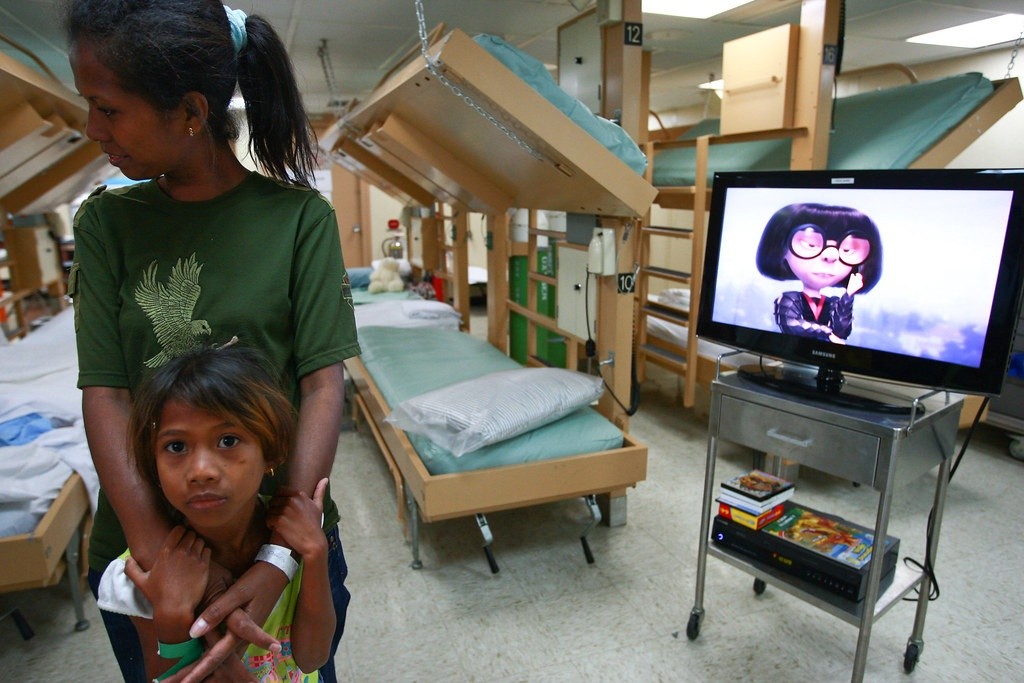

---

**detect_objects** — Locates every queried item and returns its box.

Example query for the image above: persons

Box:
[100,348,335,682]
[63,3,358,681]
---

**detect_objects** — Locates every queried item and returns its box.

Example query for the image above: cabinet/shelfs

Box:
[686,350,969,683]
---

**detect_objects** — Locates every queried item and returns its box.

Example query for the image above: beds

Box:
[308,21,661,575]
[638,61,1024,476]
[0,32,126,644]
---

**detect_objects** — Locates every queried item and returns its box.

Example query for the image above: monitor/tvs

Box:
[697,169,1024,415]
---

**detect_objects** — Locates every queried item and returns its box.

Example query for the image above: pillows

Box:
[345,268,375,289]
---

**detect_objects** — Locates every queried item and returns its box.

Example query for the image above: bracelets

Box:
[153,637,203,682]
[255,544,300,582]
